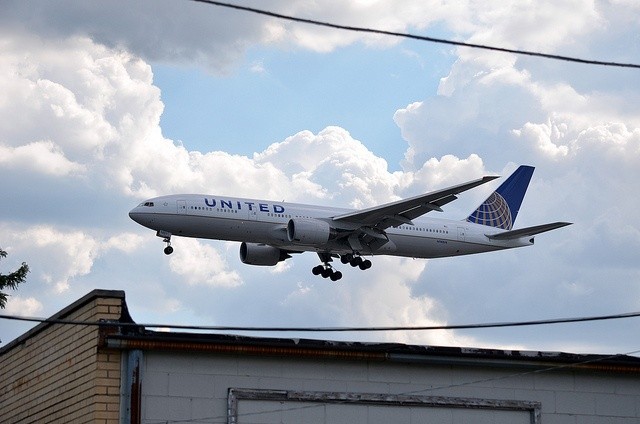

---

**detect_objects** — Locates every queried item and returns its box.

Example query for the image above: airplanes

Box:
[129,163,574,281]
